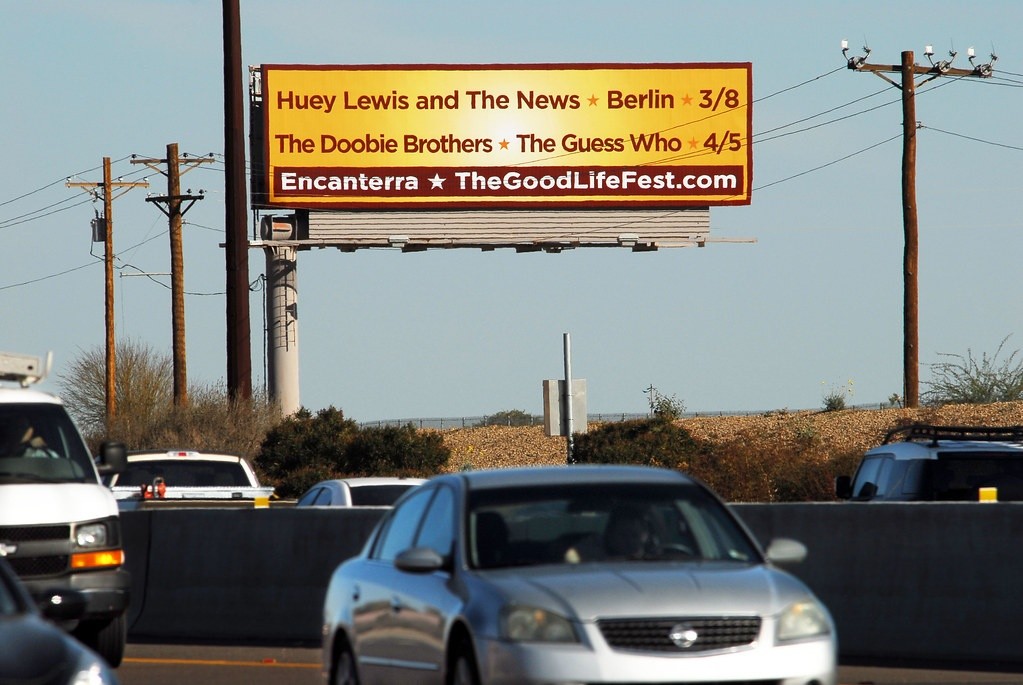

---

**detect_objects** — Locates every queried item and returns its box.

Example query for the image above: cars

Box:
[93,449,276,502]
[296,477,431,509]
[324,466,839,685]
[0,553,121,685]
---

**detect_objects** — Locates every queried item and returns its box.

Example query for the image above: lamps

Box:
[617,233,639,247]
[387,235,410,249]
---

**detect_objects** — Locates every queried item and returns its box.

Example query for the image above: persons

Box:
[0,414,59,460]
[603,506,651,560]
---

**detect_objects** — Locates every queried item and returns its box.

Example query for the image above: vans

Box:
[0,349,132,670]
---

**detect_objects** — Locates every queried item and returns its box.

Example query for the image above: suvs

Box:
[833,422,1023,502]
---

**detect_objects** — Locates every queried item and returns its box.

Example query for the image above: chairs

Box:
[602,509,657,554]
[481,506,515,562]
